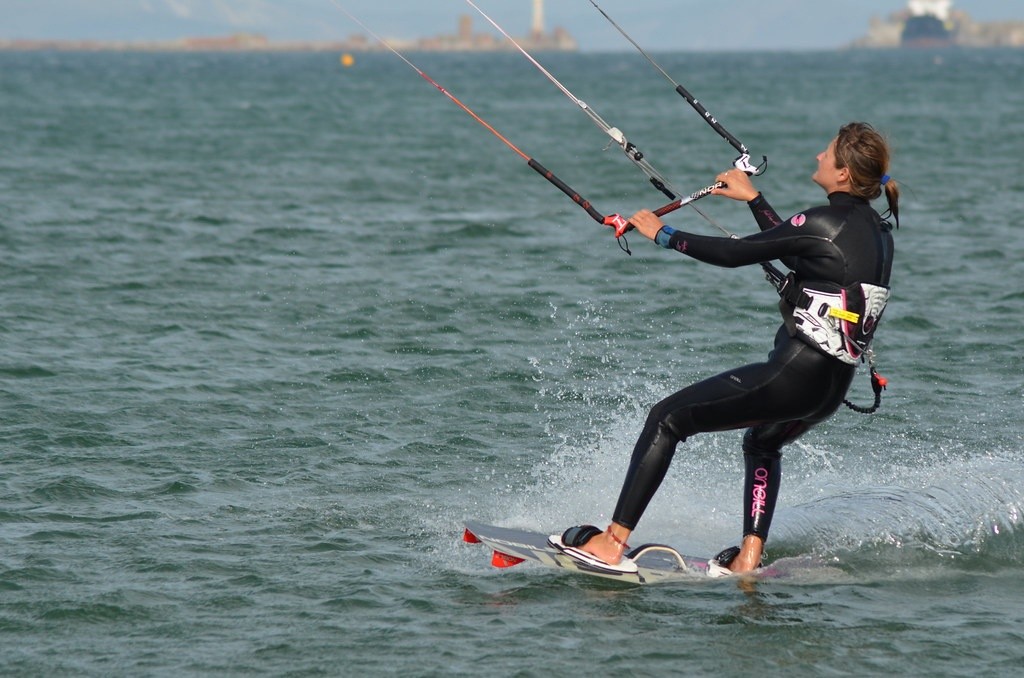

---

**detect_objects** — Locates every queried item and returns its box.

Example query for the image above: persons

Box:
[561,122,900,576]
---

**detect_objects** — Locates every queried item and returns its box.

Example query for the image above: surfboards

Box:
[462,518,765,590]
